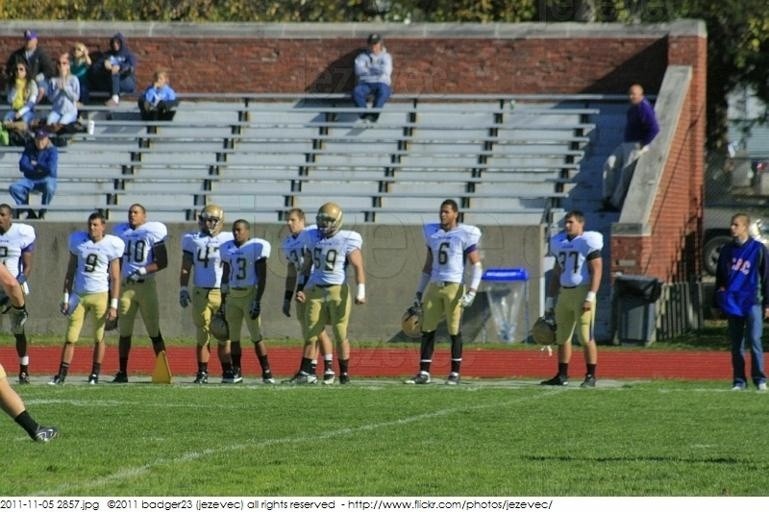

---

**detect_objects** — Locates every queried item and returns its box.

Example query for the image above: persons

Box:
[45,208,123,387]
[409,198,483,386]
[2,28,175,217]
[112,204,171,387]
[544,213,606,387]
[178,205,365,393]
[596,85,661,215]
[356,35,393,121]
[1,206,35,386]
[714,215,766,391]
[0,267,61,446]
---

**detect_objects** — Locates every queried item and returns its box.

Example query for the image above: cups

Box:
[86,120,96,136]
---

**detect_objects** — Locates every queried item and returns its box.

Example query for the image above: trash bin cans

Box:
[614,276,664,349]
[480,267,529,344]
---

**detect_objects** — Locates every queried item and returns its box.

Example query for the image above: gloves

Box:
[127,270,141,284]
[461,291,476,309]
[180,290,192,307]
[283,296,292,316]
[249,302,261,320]
[10,304,29,331]
[219,303,226,317]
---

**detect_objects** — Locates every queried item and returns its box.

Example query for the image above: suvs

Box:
[700,204,769,276]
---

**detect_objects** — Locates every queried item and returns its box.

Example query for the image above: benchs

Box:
[0,92,657,225]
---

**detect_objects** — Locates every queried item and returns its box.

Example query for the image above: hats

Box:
[24,28,38,40]
[34,129,48,139]
[366,32,381,44]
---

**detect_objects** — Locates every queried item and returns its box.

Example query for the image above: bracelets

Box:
[546,295,553,311]
[62,293,69,305]
[111,297,119,309]
[416,271,429,295]
[584,289,596,303]
[138,265,147,274]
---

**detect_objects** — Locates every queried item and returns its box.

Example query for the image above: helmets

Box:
[211,306,229,342]
[534,317,556,344]
[316,201,343,237]
[401,306,422,338]
[198,206,224,237]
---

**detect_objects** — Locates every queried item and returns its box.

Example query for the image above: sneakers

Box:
[757,383,768,391]
[19,374,30,384]
[222,368,242,383]
[48,375,64,385]
[33,422,59,445]
[263,370,274,384]
[404,369,431,384]
[581,375,596,388]
[540,373,568,386]
[113,372,128,383]
[733,382,745,391]
[445,371,461,384]
[281,370,350,385]
[89,375,98,385]
[194,369,208,384]
